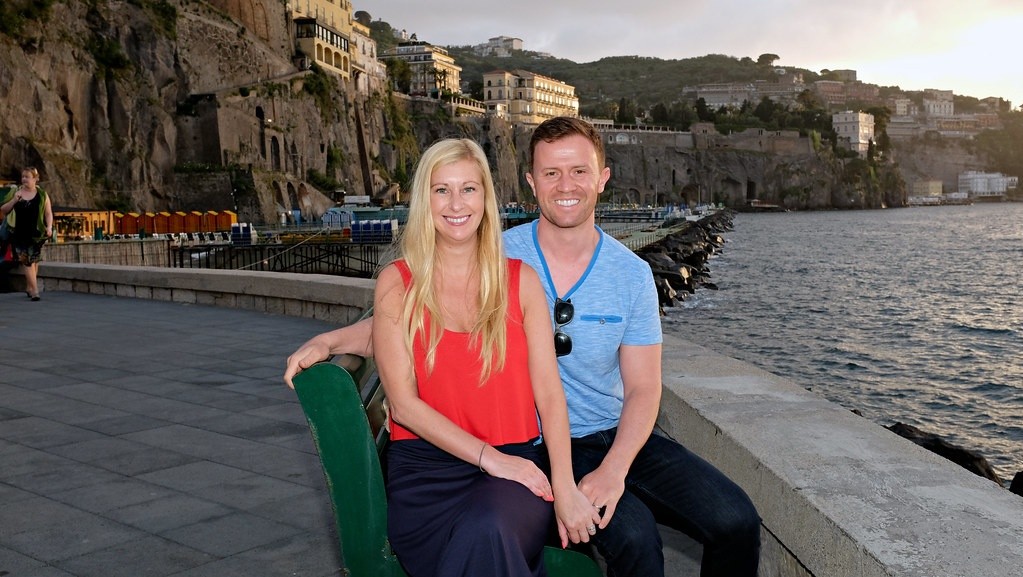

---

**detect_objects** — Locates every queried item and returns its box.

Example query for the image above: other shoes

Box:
[26,289,40,301]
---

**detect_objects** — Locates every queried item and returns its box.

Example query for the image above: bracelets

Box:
[479,442,490,474]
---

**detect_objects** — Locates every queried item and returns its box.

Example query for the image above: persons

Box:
[282,115,763,577]
[0,166,54,302]
[371,136,600,577]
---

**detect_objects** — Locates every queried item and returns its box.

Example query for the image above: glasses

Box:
[553,297,575,358]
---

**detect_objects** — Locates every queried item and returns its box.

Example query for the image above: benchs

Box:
[291,306,603,577]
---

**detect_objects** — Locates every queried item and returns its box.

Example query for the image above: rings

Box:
[593,504,602,511]
[587,526,596,531]
